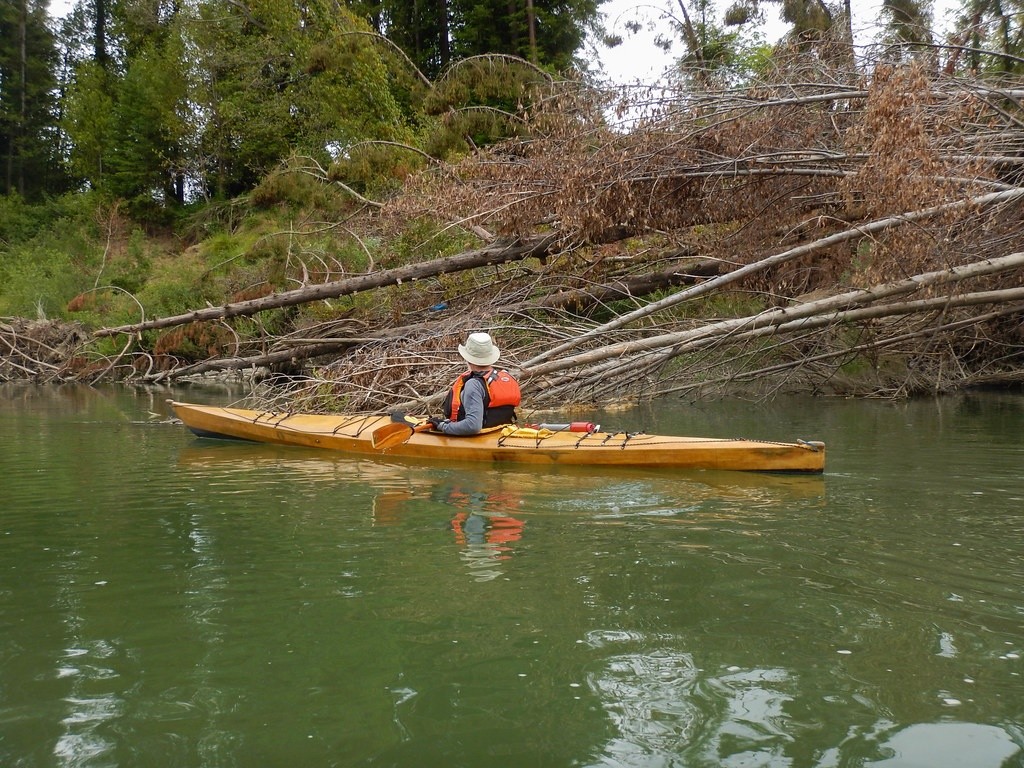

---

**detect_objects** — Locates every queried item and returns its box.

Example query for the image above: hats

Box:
[458,332,501,366]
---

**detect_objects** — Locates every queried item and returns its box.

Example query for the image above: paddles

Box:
[370,419,450,450]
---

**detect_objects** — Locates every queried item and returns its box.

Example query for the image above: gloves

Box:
[426,417,444,431]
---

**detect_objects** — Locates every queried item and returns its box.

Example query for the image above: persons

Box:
[425,333,521,435]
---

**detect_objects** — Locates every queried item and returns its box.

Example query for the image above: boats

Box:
[166,398,826,475]
